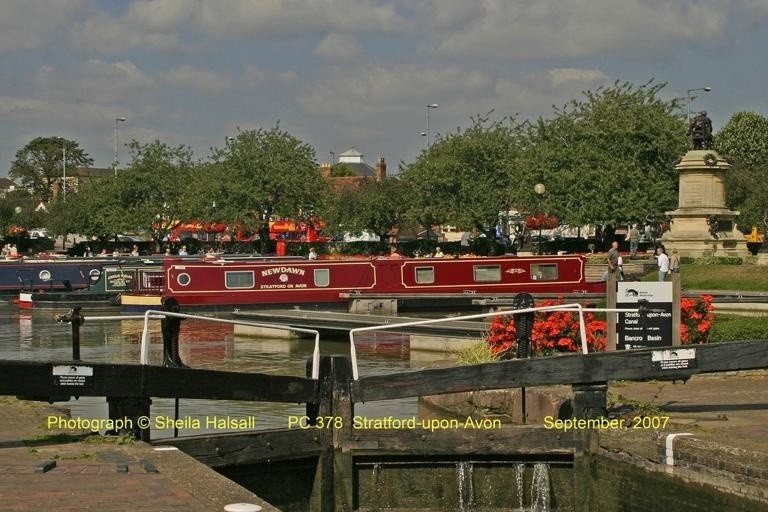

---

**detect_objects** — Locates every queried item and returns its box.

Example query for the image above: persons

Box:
[656,247,669,282]
[686,111,712,136]
[607,242,619,272]
[1,243,18,257]
[97,244,120,257]
[460,229,474,253]
[178,245,188,256]
[164,248,172,256]
[670,247,680,272]
[131,244,140,256]
[83,246,93,257]
[625,223,639,255]
[390,245,401,257]
[433,246,444,257]
[308,247,317,259]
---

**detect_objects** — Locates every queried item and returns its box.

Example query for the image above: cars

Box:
[343,228,380,243]
[532,234,652,254]
[29,230,56,243]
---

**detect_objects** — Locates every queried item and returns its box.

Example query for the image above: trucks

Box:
[468,210,528,252]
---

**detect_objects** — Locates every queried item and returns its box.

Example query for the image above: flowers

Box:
[485,295,608,355]
[680,294,715,344]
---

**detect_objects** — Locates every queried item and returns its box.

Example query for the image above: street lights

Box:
[211,200,217,253]
[686,87,711,148]
[14,206,23,254]
[420,103,438,240]
[57,136,66,250]
[228,136,235,147]
[114,117,126,180]
[534,182,545,255]
